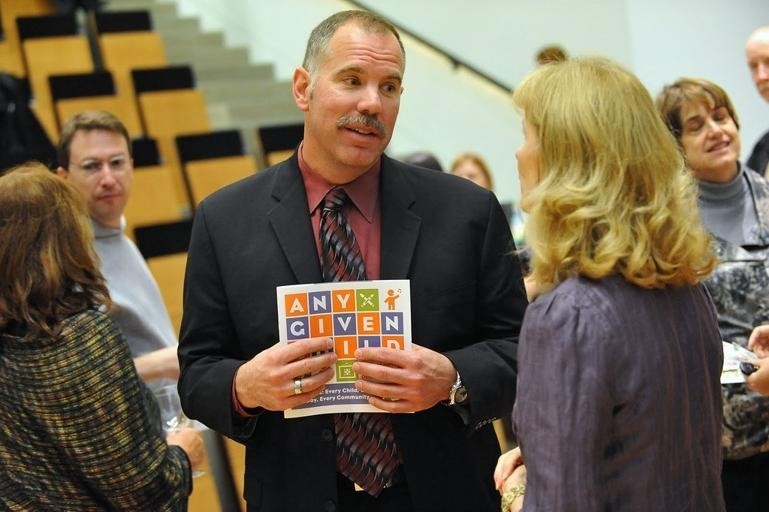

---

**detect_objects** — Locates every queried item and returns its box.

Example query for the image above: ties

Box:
[316,187,401,498]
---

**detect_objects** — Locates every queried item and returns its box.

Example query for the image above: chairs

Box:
[86,5,153,65]
[45,69,117,134]
[133,217,193,259]
[258,122,304,168]
[129,137,164,172]
[130,62,197,136]
[14,11,80,80]
[173,127,250,215]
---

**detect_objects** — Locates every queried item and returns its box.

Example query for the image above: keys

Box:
[740,362,760,375]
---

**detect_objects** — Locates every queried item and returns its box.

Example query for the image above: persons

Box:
[174,7,533,512]
[738,324,769,400]
[52,109,212,436]
[0,158,208,510]
[489,54,732,511]
[649,75,768,512]
[448,154,493,193]
[741,25,768,187]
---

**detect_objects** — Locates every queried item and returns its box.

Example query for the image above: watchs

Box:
[443,370,469,408]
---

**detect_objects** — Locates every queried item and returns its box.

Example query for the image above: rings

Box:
[294,378,303,395]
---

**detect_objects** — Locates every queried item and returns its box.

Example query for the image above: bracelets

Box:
[500,482,527,511]
